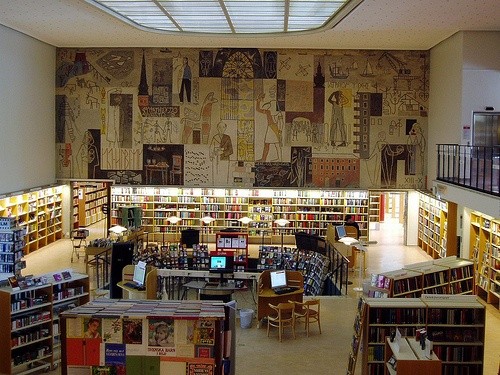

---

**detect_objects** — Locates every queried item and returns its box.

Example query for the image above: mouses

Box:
[138,288,143,291]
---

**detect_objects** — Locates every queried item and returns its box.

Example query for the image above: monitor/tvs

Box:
[133,265,147,290]
[181,230,199,248]
[336,225,346,238]
[270,270,288,291]
[211,256,227,269]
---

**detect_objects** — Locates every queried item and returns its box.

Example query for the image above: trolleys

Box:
[70,230,87,263]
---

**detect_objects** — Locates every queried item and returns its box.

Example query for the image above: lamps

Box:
[165,216,182,224]
[237,216,253,224]
[199,216,215,223]
[274,219,290,227]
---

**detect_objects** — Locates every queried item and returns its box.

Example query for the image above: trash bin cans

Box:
[240,309,255,329]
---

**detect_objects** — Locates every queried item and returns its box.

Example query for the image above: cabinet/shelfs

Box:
[0,181,500,375]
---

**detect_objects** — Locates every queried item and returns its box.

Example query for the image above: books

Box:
[427,308,484,375]
[73,181,107,228]
[110,186,369,237]
[417,192,447,258]
[368,308,425,375]
[345,296,366,375]
[9,272,84,367]
[66,299,225,375]
[0,215,26,272]
[0,183,62,243]
[366,265,472,298]
[370,192,380,222]
[471,215,500,296]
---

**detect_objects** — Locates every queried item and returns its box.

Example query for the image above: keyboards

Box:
[275,287,294,295]
[124,283,140,289]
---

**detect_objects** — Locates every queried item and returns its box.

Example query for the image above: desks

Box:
[157,268,260,303]
[117,280,146,292]
[259,287,304,297]
[183,281,248,301]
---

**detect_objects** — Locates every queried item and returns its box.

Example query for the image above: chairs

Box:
[266,303,296,342]
[287,299,322,338]
